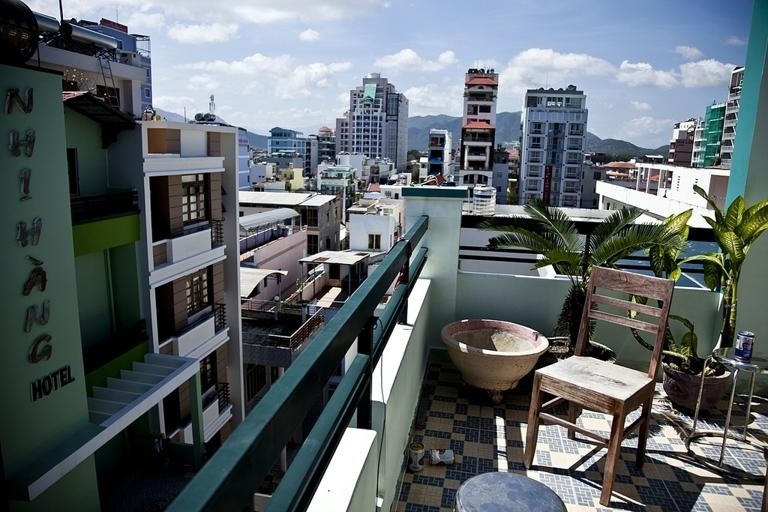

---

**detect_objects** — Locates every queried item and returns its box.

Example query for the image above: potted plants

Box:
[485,194,675,407]
[658,185,768,411]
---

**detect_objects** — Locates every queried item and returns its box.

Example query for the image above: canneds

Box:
[734,329,755,363]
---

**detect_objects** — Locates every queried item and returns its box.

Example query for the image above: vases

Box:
[442,317,550,404]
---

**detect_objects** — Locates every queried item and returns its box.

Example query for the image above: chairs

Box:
[518,262,675,506]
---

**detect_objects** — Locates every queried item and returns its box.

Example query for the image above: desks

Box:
[690,345,767,468]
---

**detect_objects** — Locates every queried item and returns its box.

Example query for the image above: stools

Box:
[454,471,568,512]
